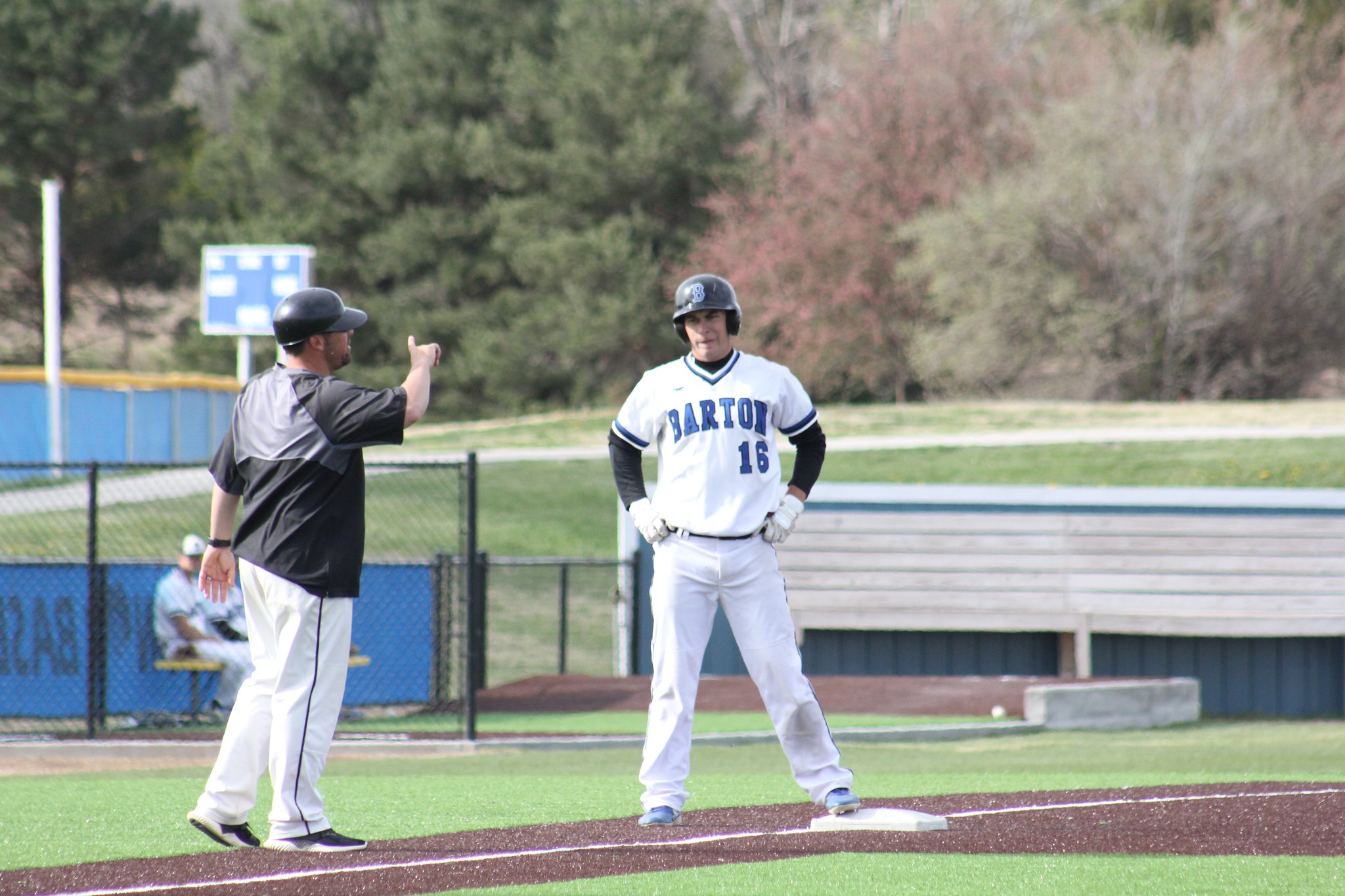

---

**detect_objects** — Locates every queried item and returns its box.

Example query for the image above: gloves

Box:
[629,498,675,544]
[753,495,805,543]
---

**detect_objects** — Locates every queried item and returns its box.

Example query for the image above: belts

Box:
[667,527,766,540]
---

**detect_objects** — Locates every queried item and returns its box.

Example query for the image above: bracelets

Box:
[207,535,231,548]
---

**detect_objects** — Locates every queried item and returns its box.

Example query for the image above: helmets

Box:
[673,274,742,345]
[272,289,368,345]
[178,535,205,555]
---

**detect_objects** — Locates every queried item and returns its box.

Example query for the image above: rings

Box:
[207,575,212,581]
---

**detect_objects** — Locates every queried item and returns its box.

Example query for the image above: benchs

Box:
[153,654,370,728]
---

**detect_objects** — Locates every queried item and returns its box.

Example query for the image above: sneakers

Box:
[825,788,861,815]
[187,811,260,847]
[263,829,369,852]
[637,806,682,827]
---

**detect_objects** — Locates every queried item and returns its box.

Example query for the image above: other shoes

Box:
[338,708,364,723]
[138,712,177,728]
[204,700,232,721]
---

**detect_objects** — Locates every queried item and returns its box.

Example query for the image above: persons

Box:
[188,286,441,852]
[154,535,254,724]
[608,274,862,827]
